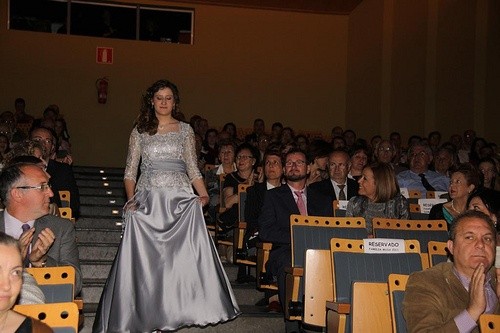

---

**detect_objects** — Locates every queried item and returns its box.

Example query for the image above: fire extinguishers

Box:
[96,76,109,104]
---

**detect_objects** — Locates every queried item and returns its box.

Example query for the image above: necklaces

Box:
[452,200,462,214]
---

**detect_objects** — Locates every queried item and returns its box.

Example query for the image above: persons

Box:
[0,98,83,333]
[176,112,500,333]
[92,80,243,333]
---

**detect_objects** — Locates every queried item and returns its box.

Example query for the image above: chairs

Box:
[200,165,500,333]
[11,189,83,333]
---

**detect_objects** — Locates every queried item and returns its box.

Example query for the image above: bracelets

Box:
[40,259,46,267]
